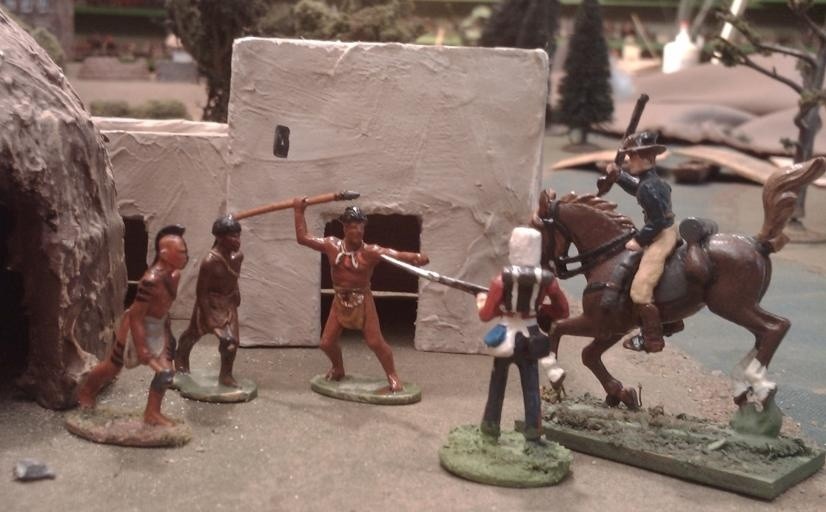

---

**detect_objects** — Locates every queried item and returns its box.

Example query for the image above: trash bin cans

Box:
[621,27,641,59]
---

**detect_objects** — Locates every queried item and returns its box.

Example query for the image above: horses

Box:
[538,156,825,411]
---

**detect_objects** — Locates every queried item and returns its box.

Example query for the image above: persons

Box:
[175,216,253,389]
[604,132,687,352]
[466,226,570,447]
[73,224,189,430]
[291,195,430,394]
